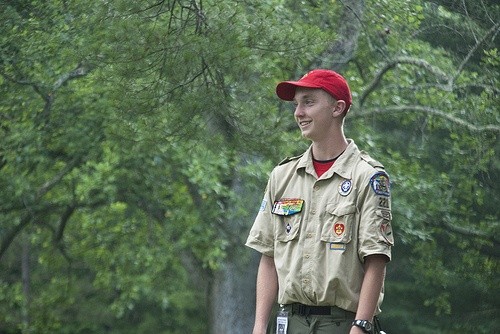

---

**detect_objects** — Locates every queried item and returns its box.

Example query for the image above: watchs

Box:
[352,319,372,333]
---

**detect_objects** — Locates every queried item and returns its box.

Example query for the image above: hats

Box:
[276,69,352,112]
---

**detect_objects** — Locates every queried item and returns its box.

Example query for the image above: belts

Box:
[292,302,332,316]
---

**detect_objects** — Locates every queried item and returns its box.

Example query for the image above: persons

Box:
[245,69,394,334]
